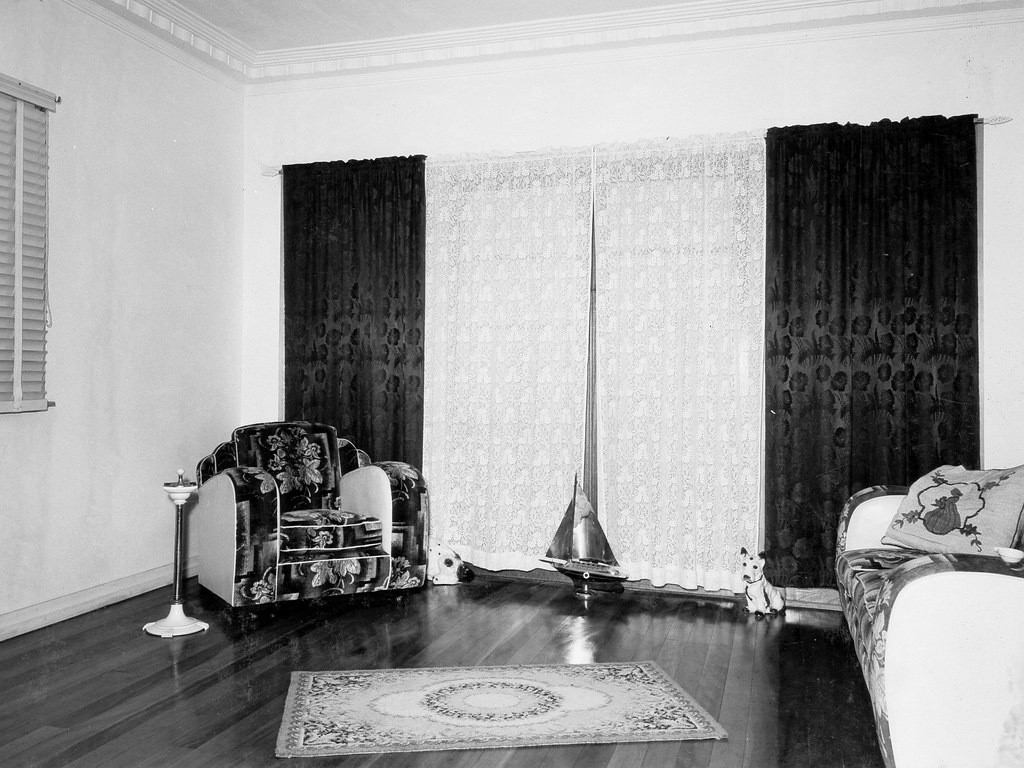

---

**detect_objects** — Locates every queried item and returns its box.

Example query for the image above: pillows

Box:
[231,420,344,515]
[881,465,1024,557]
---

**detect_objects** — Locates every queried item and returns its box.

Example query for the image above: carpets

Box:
[274,659,731,759]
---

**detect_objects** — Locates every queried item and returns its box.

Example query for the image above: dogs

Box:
[740,546,787,618]
[426,537,476,585]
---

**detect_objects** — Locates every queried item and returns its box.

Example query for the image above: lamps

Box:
[139,467,209,637]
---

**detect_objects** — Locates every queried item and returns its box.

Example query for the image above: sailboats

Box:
[538,472,629,592]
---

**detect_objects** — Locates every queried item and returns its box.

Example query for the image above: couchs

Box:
[835,463,1024,768]
[196,420,428,606]
